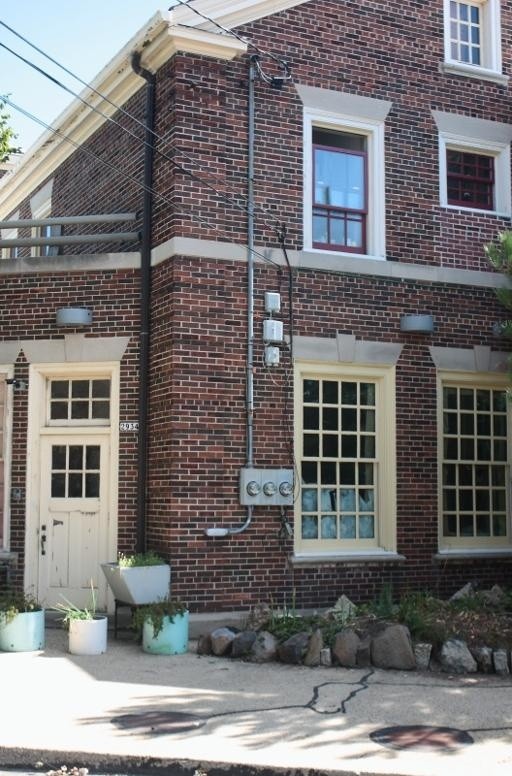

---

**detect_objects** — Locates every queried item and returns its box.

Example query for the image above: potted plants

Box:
[99,550,171,606]
[124,592,189,655]
[51,575,108,656]
[0,583,47,652]
